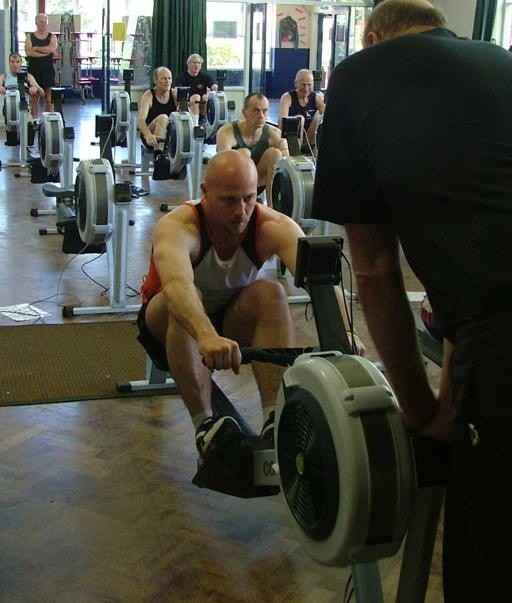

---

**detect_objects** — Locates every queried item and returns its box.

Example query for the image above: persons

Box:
[310,0,512,603]
[25,13,57,119]
[136,67,180,161]
[0,53,45,124]
[216,93,290,209]
[134,149,366,500]
[277,68,326,148]
[177,53,218,127]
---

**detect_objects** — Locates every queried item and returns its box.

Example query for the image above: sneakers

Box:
[195,414,243,457]
[125,182,148,199]
[259,413,280,444]
[153,150,168,164]
